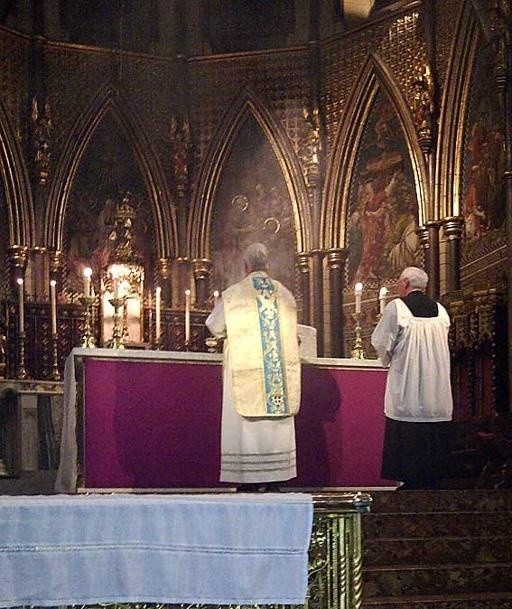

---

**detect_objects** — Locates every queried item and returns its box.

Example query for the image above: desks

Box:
[59,347,403,490]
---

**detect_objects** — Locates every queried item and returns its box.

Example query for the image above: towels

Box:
[0,492,313,609]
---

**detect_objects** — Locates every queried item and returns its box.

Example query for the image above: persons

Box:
[205,243,302,492]
[370,267,454,490]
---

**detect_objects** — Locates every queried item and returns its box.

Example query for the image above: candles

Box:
[379,287,387,314]
[50,280,57,334]
[354,282,363,313]
[17,278,24,332]
[184,290,191,341]
[114,277,121,298]
[83,268,93,298]
[155,287,162,338]
[214,291,219,308]
